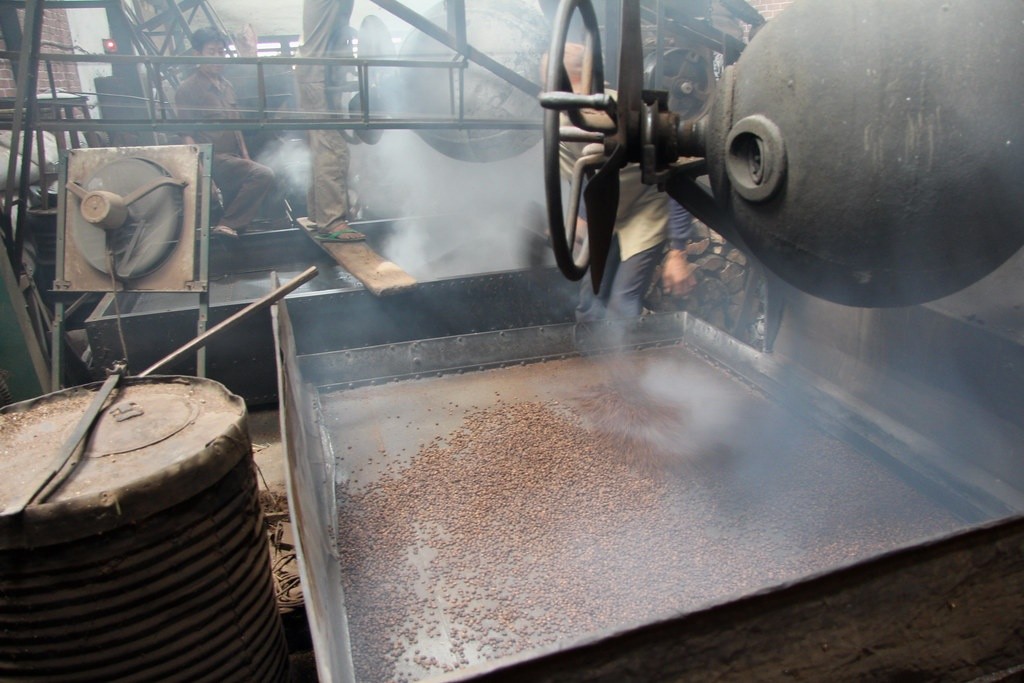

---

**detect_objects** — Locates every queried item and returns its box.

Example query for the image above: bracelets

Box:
[670,251,683,258]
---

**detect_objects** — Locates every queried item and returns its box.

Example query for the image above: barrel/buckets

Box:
[1,374,288,683]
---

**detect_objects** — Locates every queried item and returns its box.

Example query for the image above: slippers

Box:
[315,225,368,242]
[307,222,317,228]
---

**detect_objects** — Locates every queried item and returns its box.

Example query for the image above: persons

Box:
[173,27,276,241]
[542,44,695,323]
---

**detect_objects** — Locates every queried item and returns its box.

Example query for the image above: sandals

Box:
[213,224,239,240]
